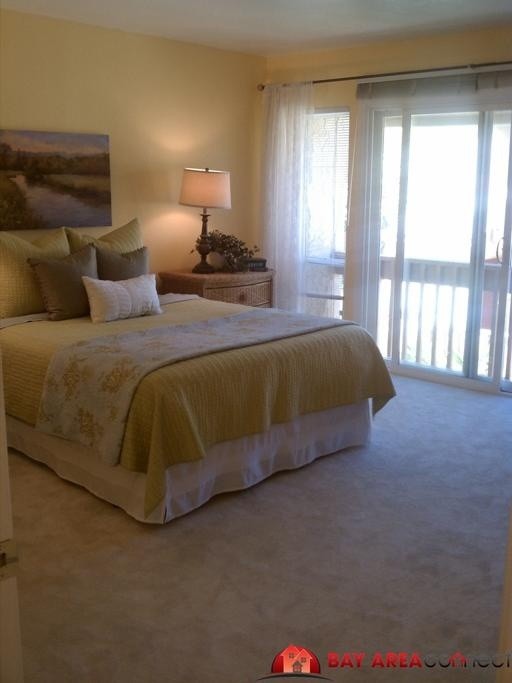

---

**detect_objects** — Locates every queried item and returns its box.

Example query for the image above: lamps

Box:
[178,168,232,273]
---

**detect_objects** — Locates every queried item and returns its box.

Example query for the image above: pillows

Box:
[0,217,162,321]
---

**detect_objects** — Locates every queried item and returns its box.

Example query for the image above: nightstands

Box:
[159,269,276,308]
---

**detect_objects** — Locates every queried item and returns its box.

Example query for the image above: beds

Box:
[0,230,398,526]
